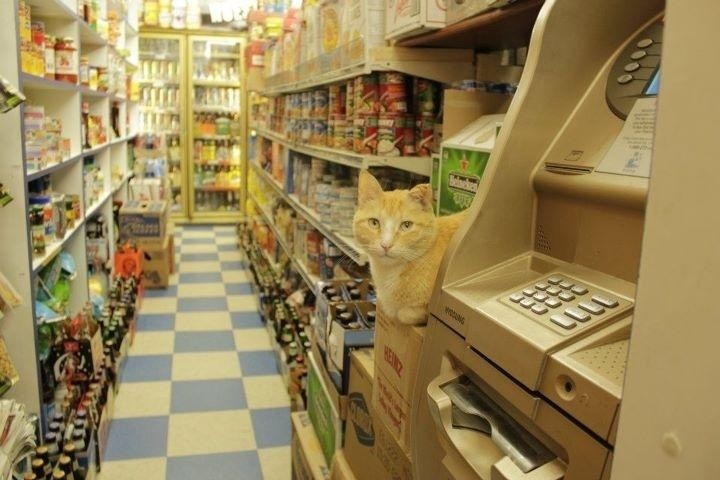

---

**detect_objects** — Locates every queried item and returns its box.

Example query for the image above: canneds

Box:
[45,34,56,81]
[28,206,45,255]
[264,72,441,157]
[314,174,359,237]
[54,38,77,83]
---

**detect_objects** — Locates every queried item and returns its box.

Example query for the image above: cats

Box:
[345,167,472,326]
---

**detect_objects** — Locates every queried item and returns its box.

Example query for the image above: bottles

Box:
[139,39,239,211]
[270,276,376,384]
[25,273,144,480]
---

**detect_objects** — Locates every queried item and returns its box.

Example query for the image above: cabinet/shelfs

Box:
[0,0,142,480]
[240,32,473,412]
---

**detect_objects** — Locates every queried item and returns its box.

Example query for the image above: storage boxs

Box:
[288,276,428,480]
[119,194,179,294]
